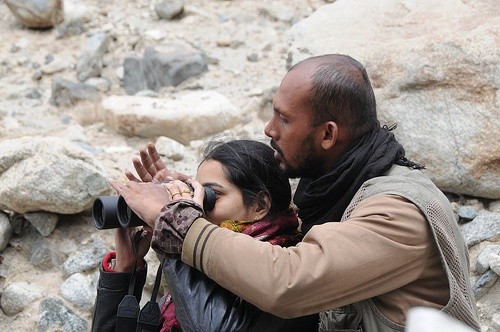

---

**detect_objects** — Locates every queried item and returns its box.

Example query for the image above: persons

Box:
[109,52,480,332]
[89,139,320,332]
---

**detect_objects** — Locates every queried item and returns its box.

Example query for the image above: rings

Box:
[180,191,191,196]
[172,192,181,199]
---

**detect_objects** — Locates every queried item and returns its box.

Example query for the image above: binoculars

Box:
[91,183,218,232]
